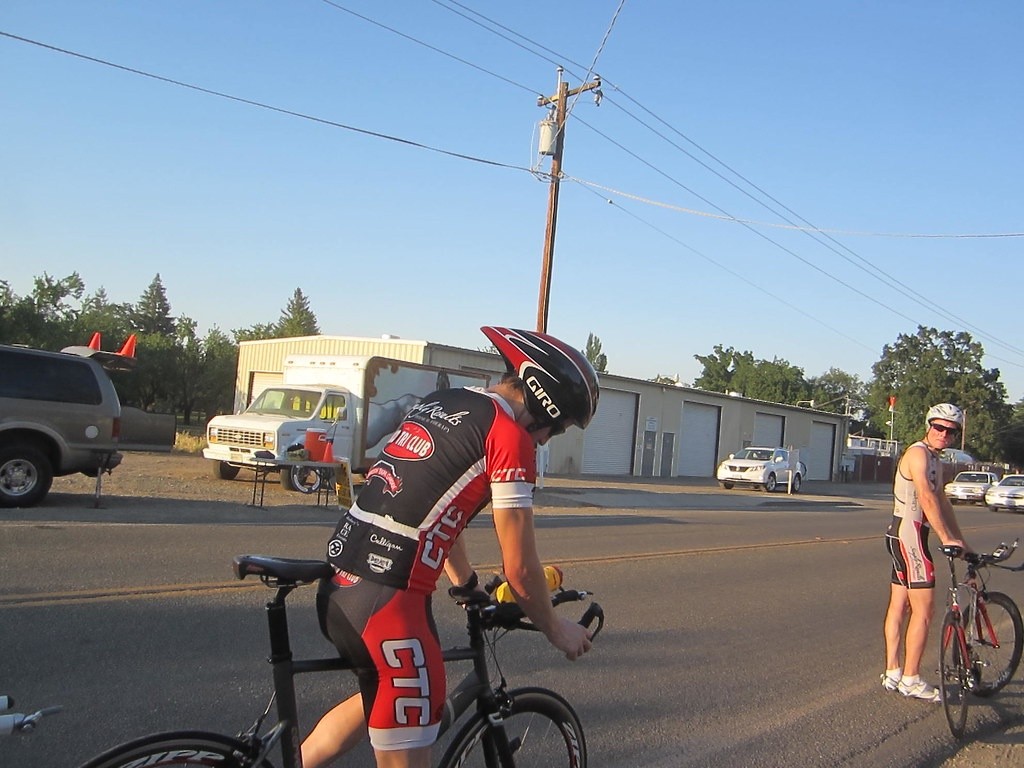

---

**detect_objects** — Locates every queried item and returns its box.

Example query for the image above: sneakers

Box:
[881,672,903,690]
[897,678,949,702]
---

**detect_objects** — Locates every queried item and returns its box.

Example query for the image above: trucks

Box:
[202,354,492,492]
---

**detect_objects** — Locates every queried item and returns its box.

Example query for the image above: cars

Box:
[944,471,998,505]
[984,475,1024,512]
[717,446,802,493]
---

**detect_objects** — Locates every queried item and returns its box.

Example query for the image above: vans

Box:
[0,345,138,509]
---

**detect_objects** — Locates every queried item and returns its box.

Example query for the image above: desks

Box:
[249,457,341,510]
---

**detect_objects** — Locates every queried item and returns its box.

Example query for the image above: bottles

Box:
[497,566,563,602]
[281,444,286,459]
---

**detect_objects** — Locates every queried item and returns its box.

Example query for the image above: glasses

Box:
[549,418,565,437]
[930,423,957,436]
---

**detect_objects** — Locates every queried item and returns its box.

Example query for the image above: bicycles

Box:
[80,555,605,768]
[937,538,1024,739]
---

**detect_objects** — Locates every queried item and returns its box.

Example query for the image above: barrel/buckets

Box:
[304,428,327,461]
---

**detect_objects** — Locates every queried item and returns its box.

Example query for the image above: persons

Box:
[879,402,975,702]
[299,324,601,767]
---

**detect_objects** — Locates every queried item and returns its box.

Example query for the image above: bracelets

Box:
[448,570,478,597]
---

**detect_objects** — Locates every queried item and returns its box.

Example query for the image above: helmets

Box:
[925,403,962,433]
[480,323,599,430]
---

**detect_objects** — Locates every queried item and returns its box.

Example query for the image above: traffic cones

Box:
[88,333,101,351]
[322,442,335,463]
[116,334,137,357]
